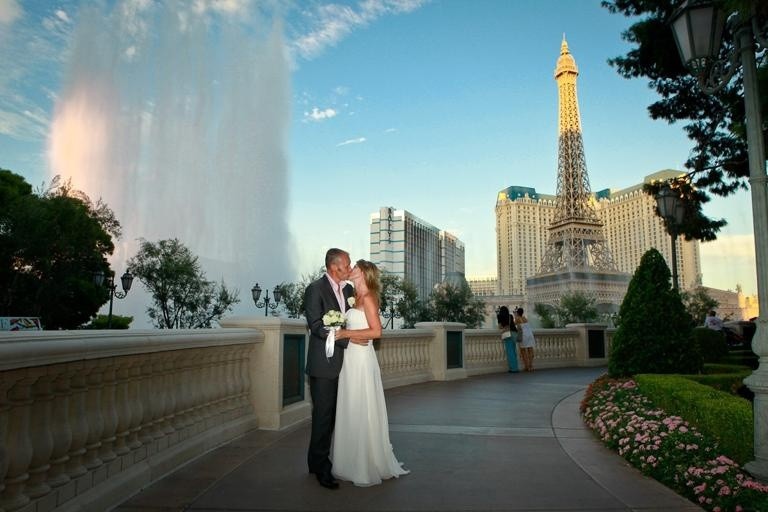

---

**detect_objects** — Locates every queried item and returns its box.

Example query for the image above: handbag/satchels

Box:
[501,331,512,340]
[517,325,523,342]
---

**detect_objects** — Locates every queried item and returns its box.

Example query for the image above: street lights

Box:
[667,1,768,481]
[252,282,283,316]
[655,185,685,292]
[611,312,621,327]
[380,298,406,329]
[495,305,518,317]
[93,268,134,328]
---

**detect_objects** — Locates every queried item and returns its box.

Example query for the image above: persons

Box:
[497,305,519,372]
[706,310,729,349]
[326,259,412,489]
[512,306,536,371]
[302,246,369,490]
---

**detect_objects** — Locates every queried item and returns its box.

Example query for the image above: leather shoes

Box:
[316,474,339,490]
